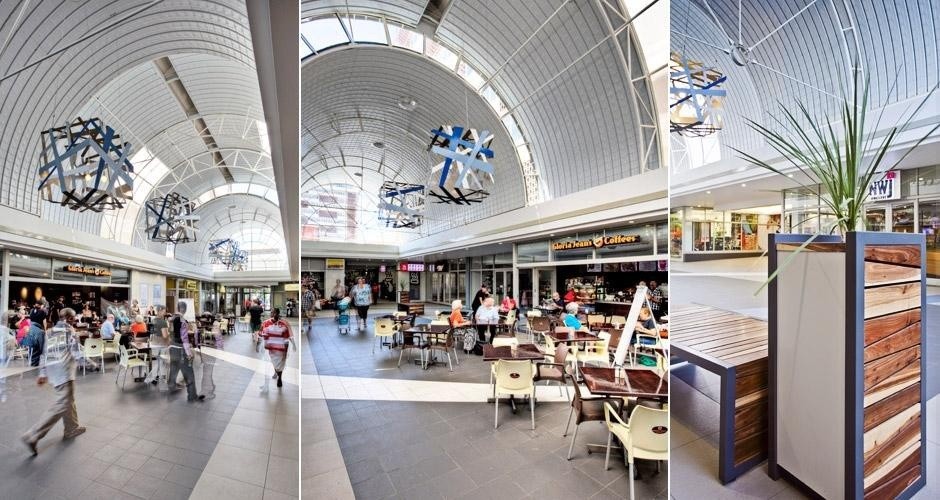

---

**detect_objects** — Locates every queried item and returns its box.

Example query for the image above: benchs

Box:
[668,302,769,487]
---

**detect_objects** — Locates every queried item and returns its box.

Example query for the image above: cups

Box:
[615,323,619,329]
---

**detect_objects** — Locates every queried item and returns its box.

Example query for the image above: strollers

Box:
[335,304,351,336]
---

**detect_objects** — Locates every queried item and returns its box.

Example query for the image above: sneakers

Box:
[276,377,282,387]
[196,394,205,400]
[22,439,39,455]
[64,426,86,439]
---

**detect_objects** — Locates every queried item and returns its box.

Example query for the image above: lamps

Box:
[208,202,249,273]
[146,131,199,246]
[38,0,134,213]
[670,0,726,137]
[377,100,425,231]
[427,34,495,204]
[0,301,256,390]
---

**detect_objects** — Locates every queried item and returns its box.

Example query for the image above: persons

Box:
[0,276,668,456]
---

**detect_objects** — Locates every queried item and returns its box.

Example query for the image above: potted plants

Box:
[723,53,939,282]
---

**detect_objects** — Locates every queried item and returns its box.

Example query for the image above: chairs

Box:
[376,296,668,500]
